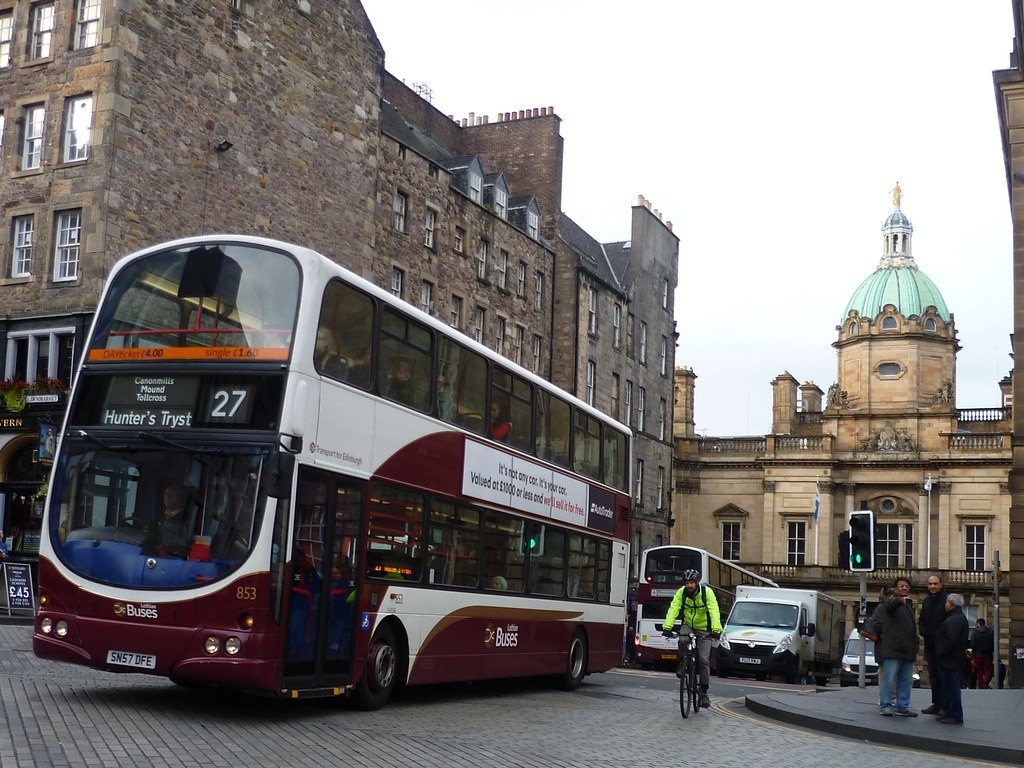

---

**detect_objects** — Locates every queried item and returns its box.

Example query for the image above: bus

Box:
[636,546,779,671]
[31,233,635,709]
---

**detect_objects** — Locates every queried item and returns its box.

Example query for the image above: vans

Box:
[840,626,879,686]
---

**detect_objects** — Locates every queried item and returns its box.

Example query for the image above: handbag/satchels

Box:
[861,619,882,641]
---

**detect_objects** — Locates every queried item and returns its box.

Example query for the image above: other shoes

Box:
[676,662,683,678]
[936,715,947,720]
[921,703,938,713]
[890,699,896,706]
[940,718,956,723]
[698,685,710,707]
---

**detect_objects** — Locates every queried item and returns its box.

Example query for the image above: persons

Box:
[870,586,897,706]
[933,594,969,725]
[880,578,919,717]
[919,574,950,715]
[889,181,903,208]
[961,651,1006,689]
[136,485,185,534]
[437,376,456,420]
[662,570,722,707]
[314,327,341,377]
[623,627,635,664]
[0,531,9,571]
[480,401,512,444]
[491,576,507,590]
[971,618,994,689]
[386,361,415,405]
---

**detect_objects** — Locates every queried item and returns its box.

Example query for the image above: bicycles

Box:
[662,631,720,719]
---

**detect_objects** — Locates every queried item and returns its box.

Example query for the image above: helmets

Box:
[686,570,700,582]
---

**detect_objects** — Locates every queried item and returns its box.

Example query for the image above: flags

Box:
[814,481,820,523]
[924,476,932,490]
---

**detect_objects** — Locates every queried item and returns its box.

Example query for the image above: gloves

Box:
[662,630,674,637]
[709,632,720,640]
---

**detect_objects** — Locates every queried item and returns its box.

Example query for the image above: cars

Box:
[911,663,921,688]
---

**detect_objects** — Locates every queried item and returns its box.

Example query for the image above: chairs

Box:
[453,412,599,480]
[333,356,350,382]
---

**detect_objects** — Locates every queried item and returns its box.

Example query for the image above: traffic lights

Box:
[849,511,875,572]
[518,518,544,556]
[837,532,849,570]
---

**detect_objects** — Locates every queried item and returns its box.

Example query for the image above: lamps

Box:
[214,140,233,153]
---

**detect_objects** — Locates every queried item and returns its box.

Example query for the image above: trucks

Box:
[716,585,846,685]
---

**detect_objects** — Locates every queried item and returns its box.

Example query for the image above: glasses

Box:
[492,408,497,411]
[929,582,938,585]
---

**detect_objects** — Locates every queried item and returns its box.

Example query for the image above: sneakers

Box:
[895,708,918,717]
[880,709,892,716]
